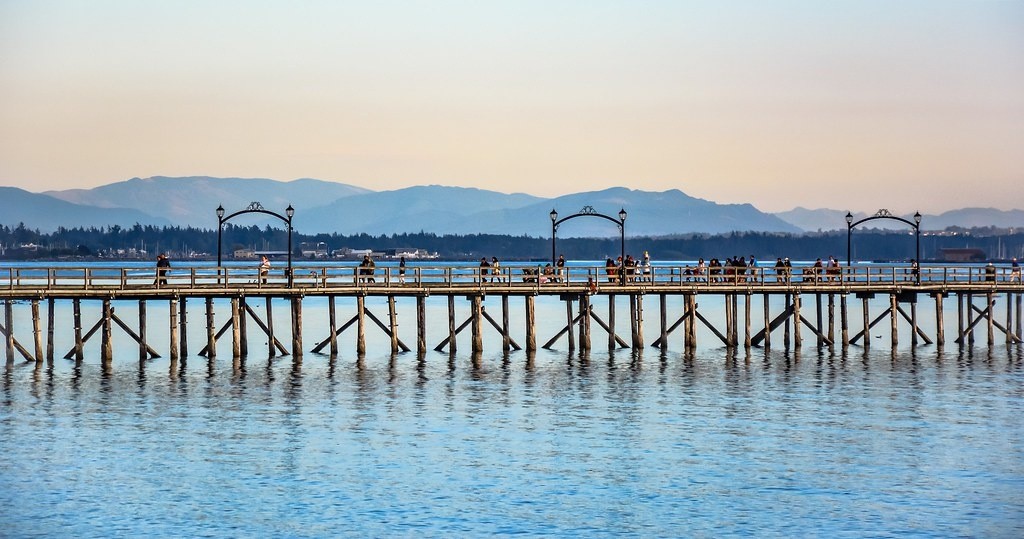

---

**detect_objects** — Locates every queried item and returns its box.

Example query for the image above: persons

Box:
[544,264,557,282]
[748,255,759,282]
[692,266,698,282]
[400,257,405,284]
[683,265,691,282]
[360,256,375,282]
[260,255,270,284]
[910,259,917,282]
[774,257,792,283]
[557,255,564,283]
[724,256,747,282]
[698,258,706,283]
[153,253,171,285]
[606,256,622,282]
[490,257,501,283]
[624,255,650,282]
[480,258,490,283]
[803,258,822,282]
[1010,257,1020,282]
[708,258,722,283]
[826,255,841,282]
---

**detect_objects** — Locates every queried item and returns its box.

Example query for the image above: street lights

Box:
[216,203,225,283]
[550,207,559,282]
[845,211,853,280]
[619,207,630,286]
[285,204,296,287]
[913,211,923,286]
